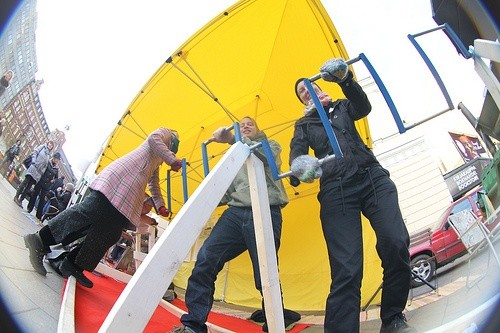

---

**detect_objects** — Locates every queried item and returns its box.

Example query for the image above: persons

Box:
[0,70,137,263]
[25,128,183,288]
[290,58,417,333]
[166,115,290,333]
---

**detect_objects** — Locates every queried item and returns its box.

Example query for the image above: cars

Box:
[406,184,498,287]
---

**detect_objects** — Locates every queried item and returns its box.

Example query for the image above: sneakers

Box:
[380,313,415,333]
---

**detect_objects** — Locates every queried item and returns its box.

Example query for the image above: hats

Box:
[53,152,61,159]
[66,183,74,191]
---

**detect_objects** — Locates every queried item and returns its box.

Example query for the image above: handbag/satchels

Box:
[23,155,32,170]
[46,241,84,278]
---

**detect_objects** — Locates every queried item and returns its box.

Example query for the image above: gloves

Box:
[158,206,169,216]
[171,159,181,172]
[290,155,323,184]
[319,57,349,82]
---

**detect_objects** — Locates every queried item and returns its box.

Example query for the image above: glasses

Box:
[299,81,315,97]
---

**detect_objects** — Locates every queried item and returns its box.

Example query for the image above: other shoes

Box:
[36,214,41,220]
[263,320,270,333]
[28,209,32,213]
[168,326,207,333]
[24,233,47,275]
[14,197,24,208]
[58,258,94,288]
